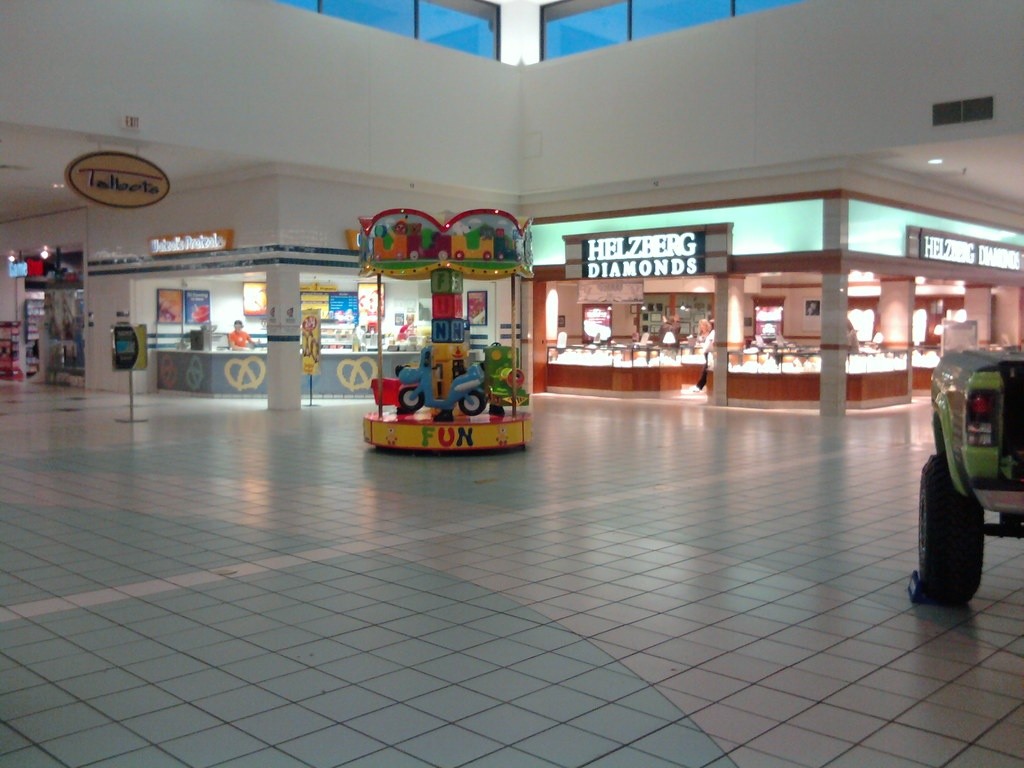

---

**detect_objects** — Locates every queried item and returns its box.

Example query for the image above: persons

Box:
[657,315,674,348]
[697,319,711,344]
[663,313,681,348]
[229,320,256,352]
[32,338,40,359]
[397,313,414,340]
[847,319,860,353]
[691,320,714,392]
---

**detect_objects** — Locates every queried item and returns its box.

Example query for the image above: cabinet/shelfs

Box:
[158,350,422,395]
[679,343,707,385]
[546,344,683,391]
[910,344,942,390]
[706,345,911,402]
